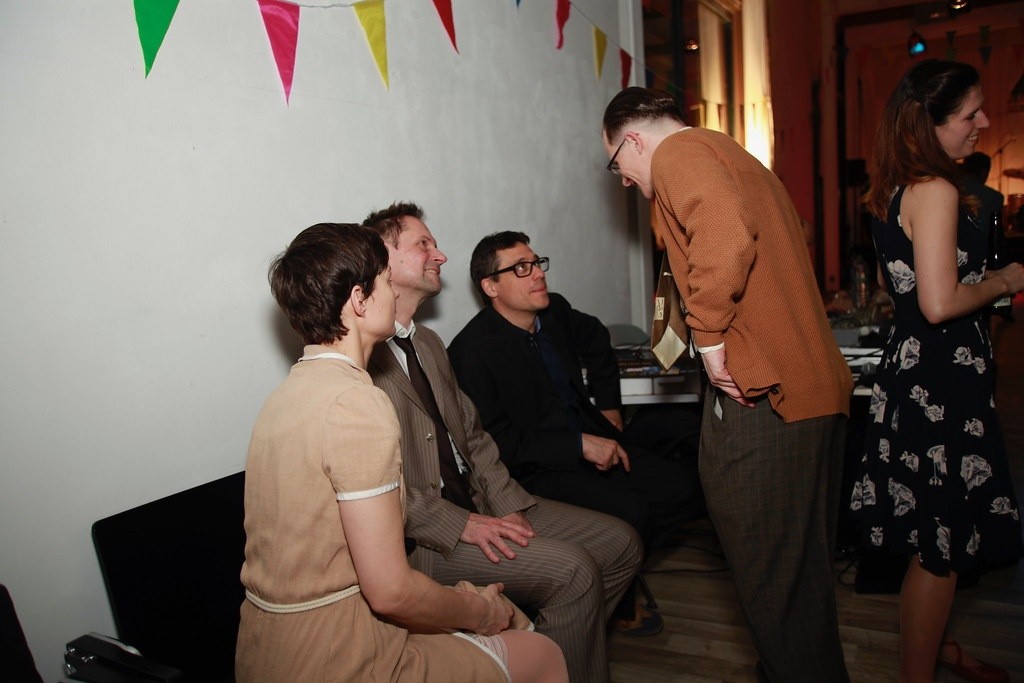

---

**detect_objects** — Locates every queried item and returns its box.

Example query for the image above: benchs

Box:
[64,470,246,683]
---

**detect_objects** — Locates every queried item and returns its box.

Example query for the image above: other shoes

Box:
[608,601,665,638]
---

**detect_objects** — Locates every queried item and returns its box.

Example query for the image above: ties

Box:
[392,332,483,515]
[651,244,688,373]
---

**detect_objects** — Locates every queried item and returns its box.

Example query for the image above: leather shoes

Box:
[937,639,1011,683]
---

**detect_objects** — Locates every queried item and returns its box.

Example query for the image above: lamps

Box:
[907,32,927,56]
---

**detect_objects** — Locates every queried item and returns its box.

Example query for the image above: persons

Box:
[446,231,664,636]
[603,87,853,683]
[846,58,1024,683]
[361,203,640,683]
[961,153,1003,265]
[234,223,569,683]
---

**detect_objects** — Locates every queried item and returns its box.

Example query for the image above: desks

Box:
[590,347,884,406]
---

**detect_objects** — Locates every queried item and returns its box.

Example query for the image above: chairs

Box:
[0,583,46,683]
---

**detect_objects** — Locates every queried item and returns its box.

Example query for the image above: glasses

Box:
[606,133,640,177]
[488,257,549,278]
